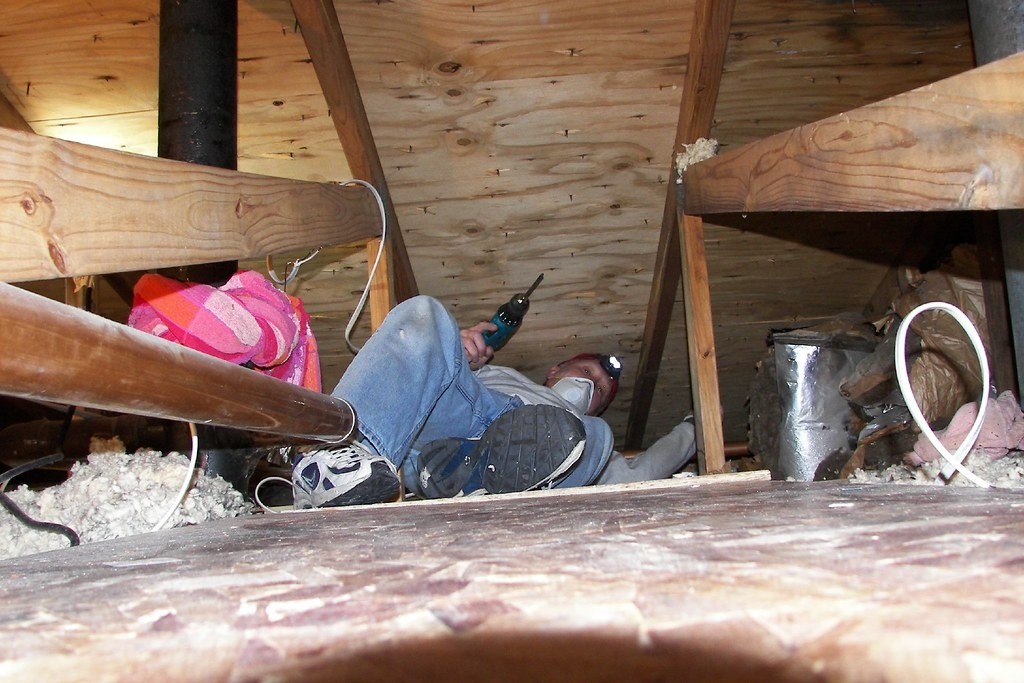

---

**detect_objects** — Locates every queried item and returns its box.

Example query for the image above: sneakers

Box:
[291,443,401,509]
[417,404,587,500]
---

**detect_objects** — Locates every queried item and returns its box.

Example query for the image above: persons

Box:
[291,296,701,509]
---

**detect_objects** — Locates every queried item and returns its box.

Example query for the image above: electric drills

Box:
[478,274,543,352]
